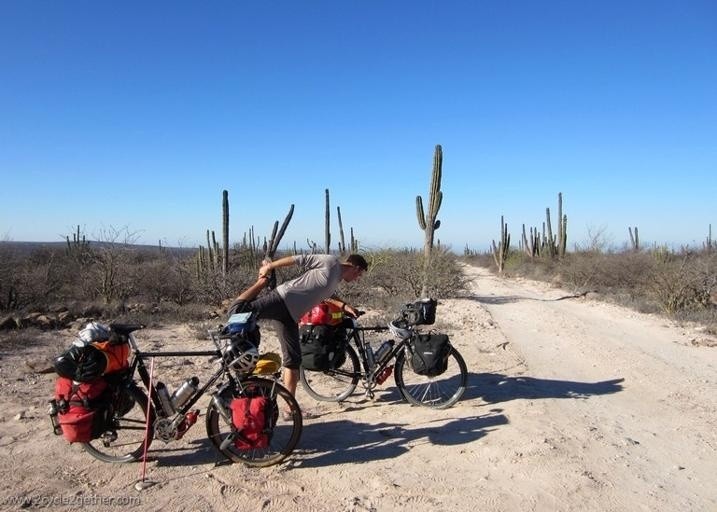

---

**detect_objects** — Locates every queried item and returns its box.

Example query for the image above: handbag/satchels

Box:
[411,333,449,377]
[55,321,133,442]
[299,302,358,372]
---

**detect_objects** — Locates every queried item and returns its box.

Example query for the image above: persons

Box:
[224,251,369,420]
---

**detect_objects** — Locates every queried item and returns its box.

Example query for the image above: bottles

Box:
[154,381,175,417]
[376,365,394,385]
[364,341,377,369]
[169,375,199,411]
[372,338,395,366]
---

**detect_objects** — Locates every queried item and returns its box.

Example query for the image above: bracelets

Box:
[341,302,348,311]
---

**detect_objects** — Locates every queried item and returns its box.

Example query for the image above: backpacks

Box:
[230,395,271,450]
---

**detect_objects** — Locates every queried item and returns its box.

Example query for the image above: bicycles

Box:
[55,310,304,476]
[292,298,469,410]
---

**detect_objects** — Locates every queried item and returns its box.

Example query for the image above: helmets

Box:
[387,318,412,340]
[221,338,259,375]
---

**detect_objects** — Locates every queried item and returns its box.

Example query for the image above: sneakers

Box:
[260,256,276,289]
[283,409,318,421]
